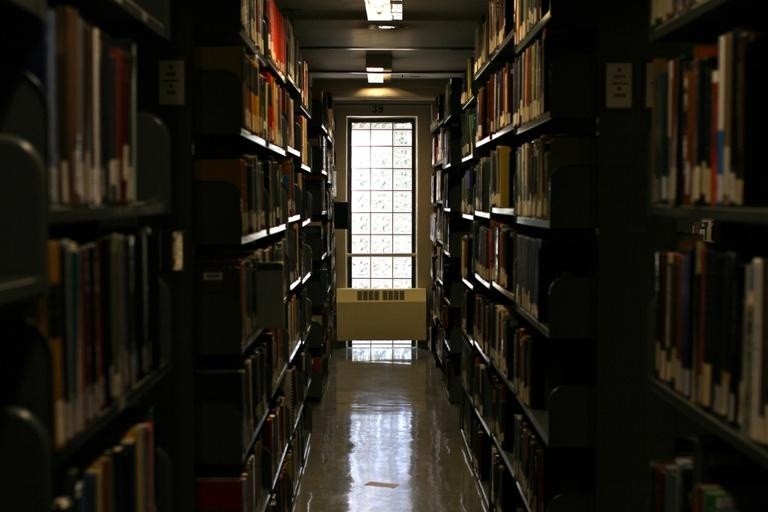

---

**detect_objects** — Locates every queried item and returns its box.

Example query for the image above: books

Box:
[428,2,767,512]
[2,0,338,511]
